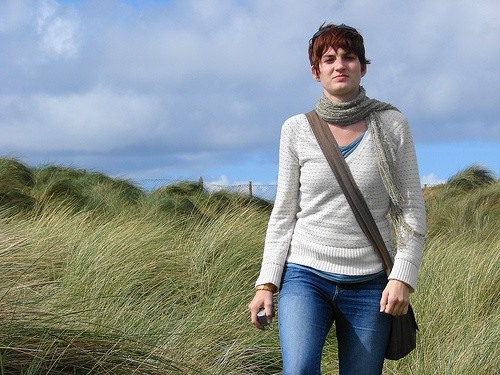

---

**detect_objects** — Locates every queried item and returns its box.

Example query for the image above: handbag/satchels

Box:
[385,303,420,360]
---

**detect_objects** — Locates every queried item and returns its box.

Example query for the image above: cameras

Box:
[257,304,275,326]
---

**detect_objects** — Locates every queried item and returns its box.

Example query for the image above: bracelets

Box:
[254,283,278,294]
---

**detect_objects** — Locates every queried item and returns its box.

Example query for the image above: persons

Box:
[247,21,429,375]
